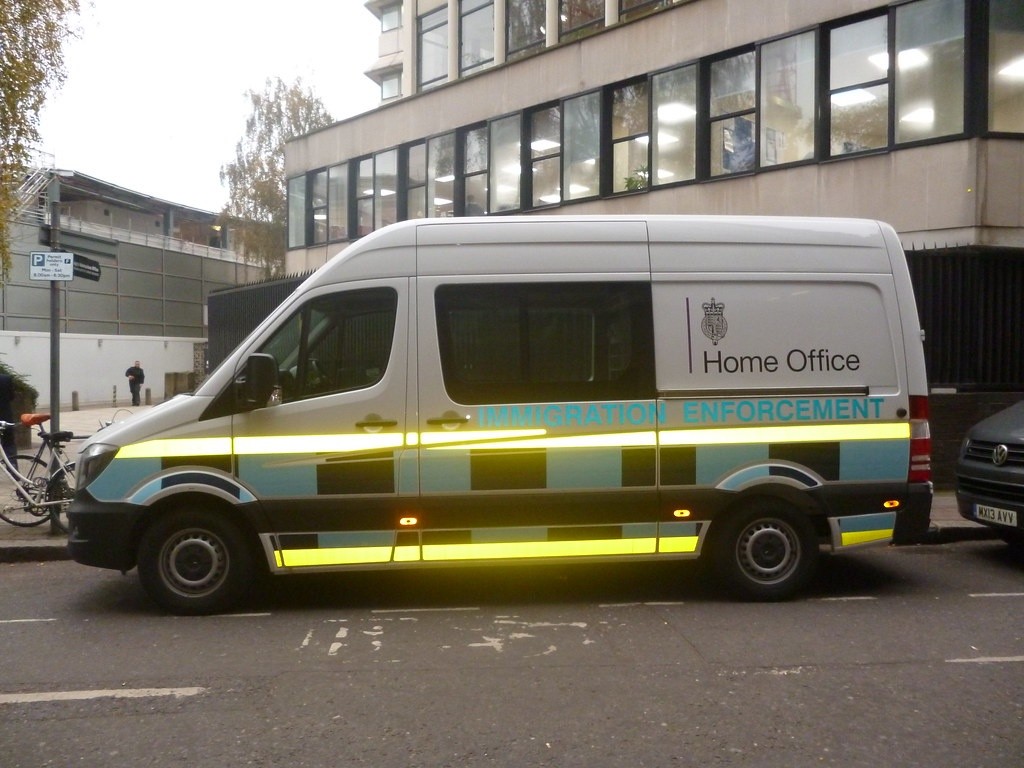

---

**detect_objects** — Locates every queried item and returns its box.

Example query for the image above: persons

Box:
[125,360,145,406]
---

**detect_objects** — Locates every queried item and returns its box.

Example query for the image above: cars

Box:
[956,402,1024,546]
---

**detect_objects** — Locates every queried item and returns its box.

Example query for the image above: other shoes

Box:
[132,402,135,406]
[136,401,139,405]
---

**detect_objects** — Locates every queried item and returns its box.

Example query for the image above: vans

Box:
[69,216,933,614]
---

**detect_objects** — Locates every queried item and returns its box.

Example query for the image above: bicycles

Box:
[0,409,139,531]
